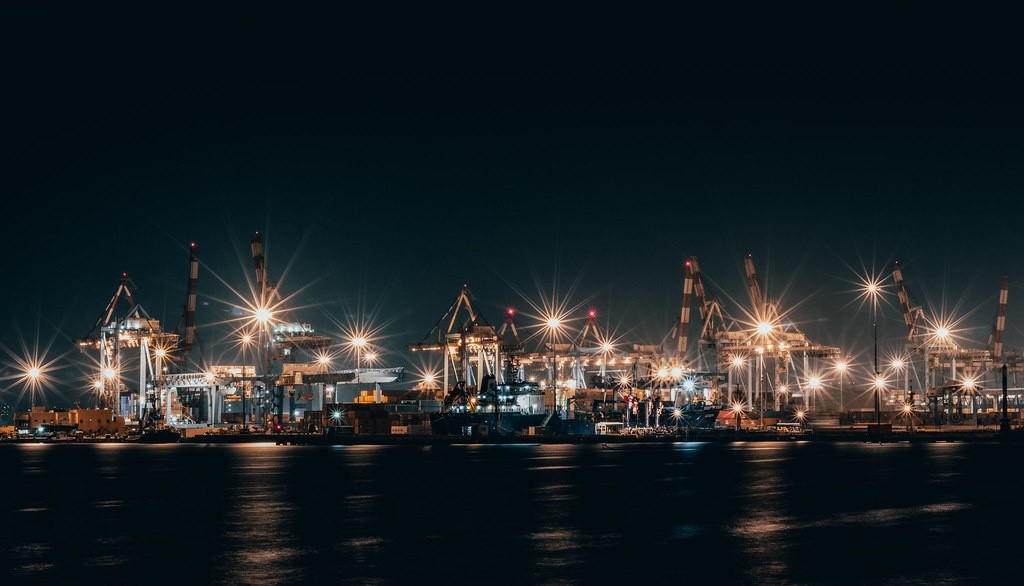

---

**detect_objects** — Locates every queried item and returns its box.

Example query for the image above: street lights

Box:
[779,386,787,411]
[758,347,765,427]
[812,379,820,411]
[319,355,332,385]
[28,367,39,429]
[735,404,741,431]
[257,308,269,431]
[240,334,249,431]
[96,382,100,408]
[735,358,745,402]
[546,318,561,415]
[353,338,366,427]
[797,411,804,428]
[602,341,612,402]
[758,321,771,411]
[838,364,845,412]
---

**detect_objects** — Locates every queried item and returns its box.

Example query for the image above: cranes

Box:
[73,280,182,412]
[406,282,528,355]
